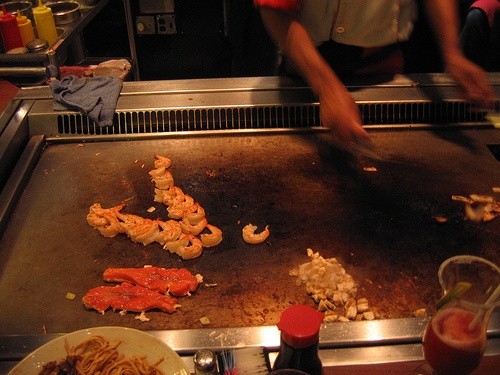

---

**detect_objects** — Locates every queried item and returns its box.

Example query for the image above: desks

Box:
[0,0,109,86]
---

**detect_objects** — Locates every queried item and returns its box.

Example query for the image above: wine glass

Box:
[413,255,500,375]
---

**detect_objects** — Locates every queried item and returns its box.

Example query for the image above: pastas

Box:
[63,336,166,375]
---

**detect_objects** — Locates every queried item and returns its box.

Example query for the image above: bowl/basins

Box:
[44,1,81,25]
[0,1,33,19]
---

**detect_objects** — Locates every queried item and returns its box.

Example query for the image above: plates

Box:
[7,326,190,375]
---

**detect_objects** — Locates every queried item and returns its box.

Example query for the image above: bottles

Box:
[32,0,59,47]
[15,9,35,48]
[26,38,49,53]
[271,304,326,375]
[193,348,219,375]
[0,4,25,54]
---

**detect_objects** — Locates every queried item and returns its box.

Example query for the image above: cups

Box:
[266,369,309,375]
[6,47,30,55]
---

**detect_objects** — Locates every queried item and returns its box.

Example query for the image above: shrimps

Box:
[241,223,270,245]
[86,150,223,262]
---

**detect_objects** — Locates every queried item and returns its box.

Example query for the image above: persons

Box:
[254,0,500,157]
